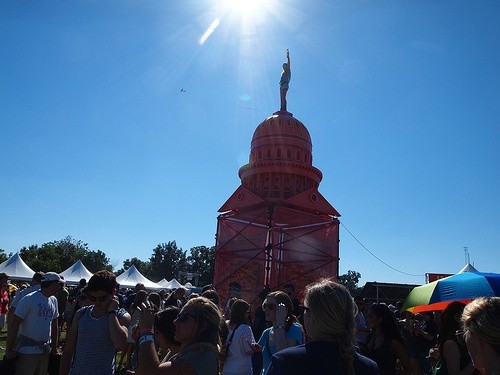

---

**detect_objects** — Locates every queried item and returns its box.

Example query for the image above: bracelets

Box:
[107,309,116,318]
[136,327,156,342]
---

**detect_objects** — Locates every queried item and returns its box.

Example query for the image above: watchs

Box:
[274,322,285,332]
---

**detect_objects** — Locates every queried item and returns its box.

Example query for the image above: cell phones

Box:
[245,339,255,351]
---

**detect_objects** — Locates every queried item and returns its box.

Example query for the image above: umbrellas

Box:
[404,271,500,316]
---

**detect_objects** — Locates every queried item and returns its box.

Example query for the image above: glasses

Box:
[176,313,194,321]
[262,302,274,310]
[89,295,108,301]
[367,313,379,319]
[355,302,363,305]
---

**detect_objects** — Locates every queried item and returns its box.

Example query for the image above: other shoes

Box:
[0,346,6,351]
[117,365,122,371]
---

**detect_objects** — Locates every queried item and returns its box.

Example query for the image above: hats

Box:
[0,273,9,280]
[41,271,65,283]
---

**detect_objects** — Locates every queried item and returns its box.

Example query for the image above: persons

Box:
[273,48,292,113]
[0,266,500,375]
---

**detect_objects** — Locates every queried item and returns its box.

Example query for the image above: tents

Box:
[166,277,187,291]
[156,278,169,288]
[114,264,161,294]
[56,259,94,286]
[0,251,39,280]
